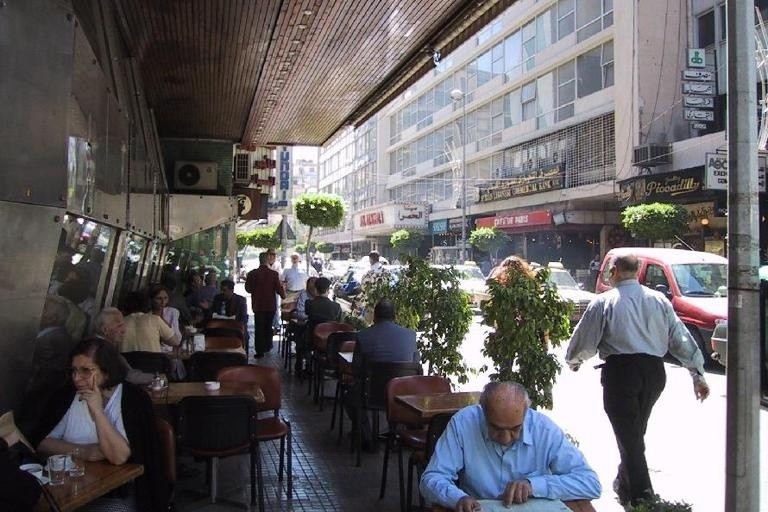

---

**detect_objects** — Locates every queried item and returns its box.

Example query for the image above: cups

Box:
[51,454,71,467]
[67,443,87,479]
[20,462,43,482]
[47,455,65,487]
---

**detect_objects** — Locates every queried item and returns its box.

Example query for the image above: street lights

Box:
[449,88,467,264]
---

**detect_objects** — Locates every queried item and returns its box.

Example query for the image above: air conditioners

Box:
[233,151,251,184]
[171,161,219,192]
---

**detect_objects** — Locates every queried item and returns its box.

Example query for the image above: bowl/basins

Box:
[204,381,222,390]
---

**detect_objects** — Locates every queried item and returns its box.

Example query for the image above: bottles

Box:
[221,302,226,316]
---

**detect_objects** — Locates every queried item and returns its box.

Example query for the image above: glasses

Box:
[65,367,96,376]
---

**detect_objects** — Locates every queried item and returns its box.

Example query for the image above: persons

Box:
[92,307,203,479]
[119,291,181,353]
[280,253,310,322]
[30,338,154,480]
[245,252,286,358]
[159,276,192,326]
[199,280,249,348]
[418,380,601,512]
[565,254,710,512]
[151,286,182,353]
[494,256,537,411]
[342,301,417,454]
[263,251,285,334]
[49,248,104,303]
[294,277,330,379]
[362,252,385,294]
[304,277,342,378]
[185,270,221,313]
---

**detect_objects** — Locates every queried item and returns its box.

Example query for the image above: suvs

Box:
[595,246,729,370]
[478,262,595,333]
[76,217,98,253]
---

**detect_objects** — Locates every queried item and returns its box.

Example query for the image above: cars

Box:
[711,321,727,368]
[429,265,486,312]
[94,232,107,251]
[238,241,410,303]
[190,265,230,281]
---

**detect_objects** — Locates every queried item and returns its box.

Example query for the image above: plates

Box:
[44,462,77,472]
[41,476,50,485]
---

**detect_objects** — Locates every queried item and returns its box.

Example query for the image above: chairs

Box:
[0,301,486,512]
